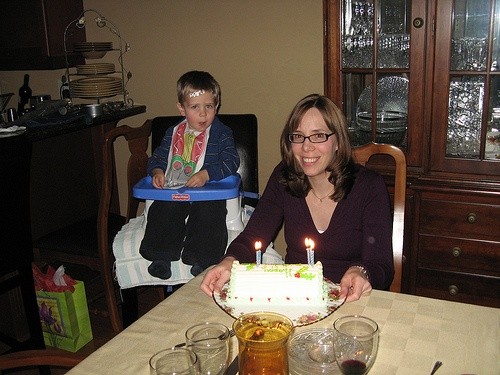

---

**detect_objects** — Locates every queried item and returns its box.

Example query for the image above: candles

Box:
[255,240,262,265]
[305,237,315,266]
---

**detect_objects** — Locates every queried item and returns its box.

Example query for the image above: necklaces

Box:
[309,185,334,203]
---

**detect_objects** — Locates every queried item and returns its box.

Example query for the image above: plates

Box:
[287,327,365,375]
[69,77,123,99]
[77,63,115,77]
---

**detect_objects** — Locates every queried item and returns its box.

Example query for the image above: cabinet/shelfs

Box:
[322,0,500,308]
[0,0,86,72]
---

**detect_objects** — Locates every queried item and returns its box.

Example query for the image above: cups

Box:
[149,347,200,375]
[185,322,230,375]
[7,108,17,122]
[333,316,379,375]
[232,312,294,375]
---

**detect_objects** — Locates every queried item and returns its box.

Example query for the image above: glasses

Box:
[287,130,335,144]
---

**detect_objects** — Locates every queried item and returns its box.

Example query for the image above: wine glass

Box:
[341,0,409,68]
[450,37,498,71]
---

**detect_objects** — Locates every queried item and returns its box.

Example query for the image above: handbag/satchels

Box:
[36,280,93,353]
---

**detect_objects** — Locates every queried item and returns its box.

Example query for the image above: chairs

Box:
[30,120,153,333]
[114,113,262,324]
[348,141,406,295]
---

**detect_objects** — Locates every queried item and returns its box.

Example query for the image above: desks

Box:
[68,264,500,375]
[0,104,146,375]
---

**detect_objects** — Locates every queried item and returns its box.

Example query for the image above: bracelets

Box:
[349,265,370,280]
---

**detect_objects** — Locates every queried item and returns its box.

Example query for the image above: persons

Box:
[139,71,241,279]
[200,92,396,311]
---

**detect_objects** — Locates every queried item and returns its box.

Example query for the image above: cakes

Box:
[226,259,327,316]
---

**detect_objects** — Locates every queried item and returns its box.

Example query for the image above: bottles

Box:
[17,74,32,116]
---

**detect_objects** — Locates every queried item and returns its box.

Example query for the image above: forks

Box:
[175,329,235,347]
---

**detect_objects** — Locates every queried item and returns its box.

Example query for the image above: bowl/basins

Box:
[71,42,112,59]
[31,95,51,103]
[355,111,407,147]
[80,104,103,118]
